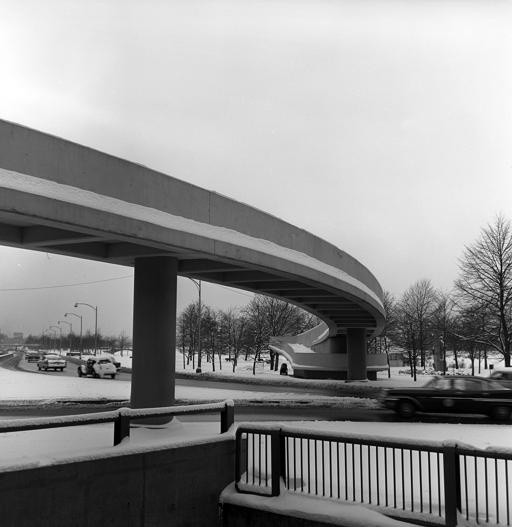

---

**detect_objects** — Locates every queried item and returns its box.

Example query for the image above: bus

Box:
[64,351,82,359]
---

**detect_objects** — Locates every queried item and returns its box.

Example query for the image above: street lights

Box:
[42,319,73,352]
[63,311,83,360]
[73,301,97,358]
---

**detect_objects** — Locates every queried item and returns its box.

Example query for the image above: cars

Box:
[75,355,118,380]
[35,354,67,372]
[0,349,8,354]
[380,375,512,423]
[484,365,512,387]
[22,348,61,364]
[99,353,122,370]
